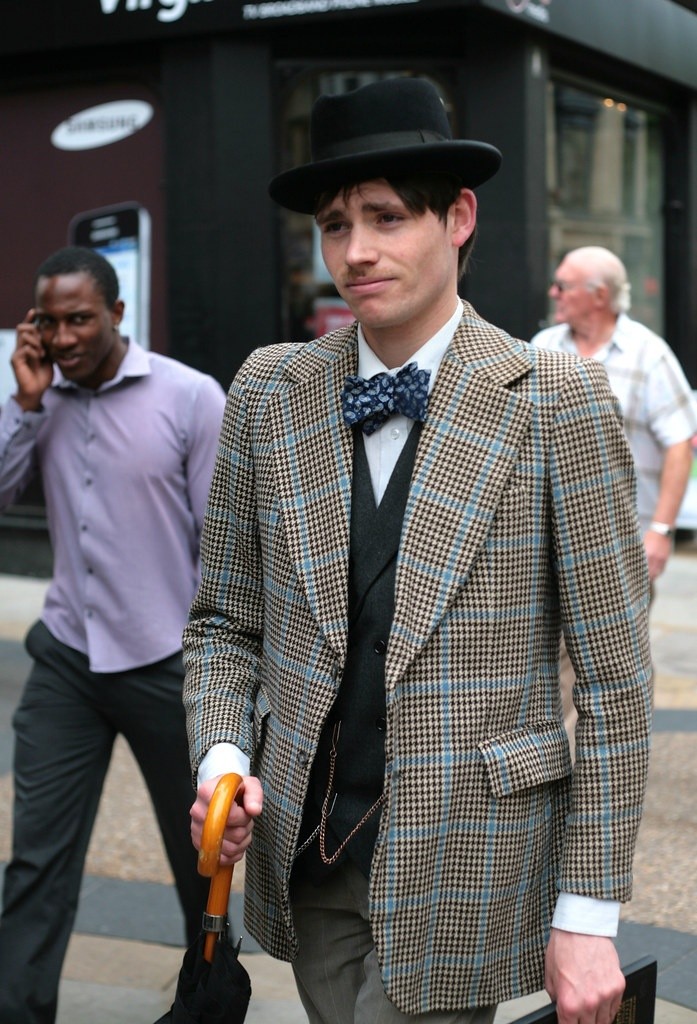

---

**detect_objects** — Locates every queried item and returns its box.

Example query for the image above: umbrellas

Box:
[168,772,252,1024]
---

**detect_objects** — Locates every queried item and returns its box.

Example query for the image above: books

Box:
[507,954,657,1024]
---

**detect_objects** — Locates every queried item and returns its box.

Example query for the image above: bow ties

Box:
[338,361,431,436]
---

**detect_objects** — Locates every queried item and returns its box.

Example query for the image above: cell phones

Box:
[68,202,149,352]
[33,316,54,368]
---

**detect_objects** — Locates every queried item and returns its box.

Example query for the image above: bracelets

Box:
[649,521,676,538]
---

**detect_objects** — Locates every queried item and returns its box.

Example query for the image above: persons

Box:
[528,247,697,727]
[0,250,254,1024]
[180,77,655,1024]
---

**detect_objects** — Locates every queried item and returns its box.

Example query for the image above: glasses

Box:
[549,276,568,292]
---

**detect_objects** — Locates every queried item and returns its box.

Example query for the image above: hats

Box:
[265,77,502,216]
